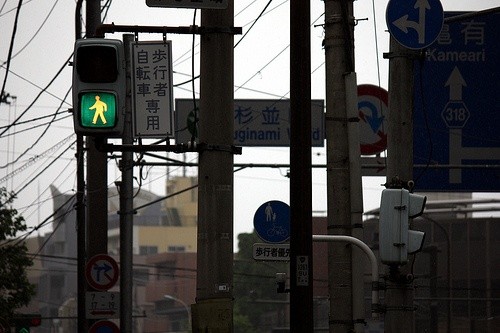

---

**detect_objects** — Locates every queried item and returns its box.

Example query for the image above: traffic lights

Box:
[379,189,426,264]
[74,39,127,139]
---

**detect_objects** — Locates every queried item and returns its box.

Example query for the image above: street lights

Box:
[164,294,191,333]
